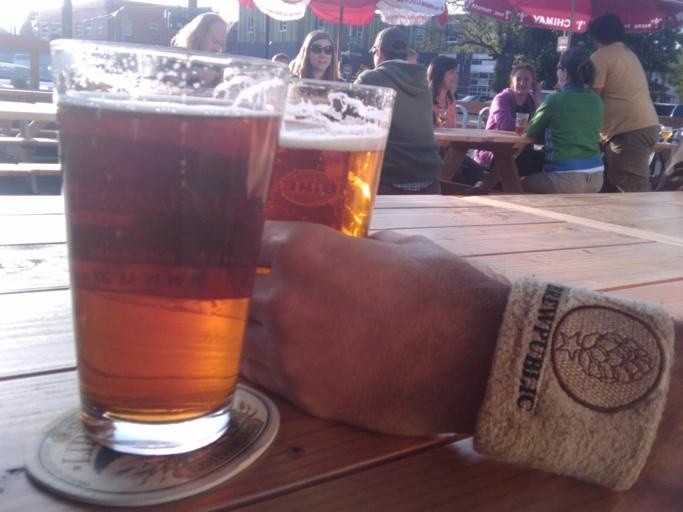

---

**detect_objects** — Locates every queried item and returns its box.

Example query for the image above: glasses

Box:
[310,44,332,54]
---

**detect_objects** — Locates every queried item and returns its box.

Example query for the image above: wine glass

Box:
[437,109,448,132]
[661,126,673,144]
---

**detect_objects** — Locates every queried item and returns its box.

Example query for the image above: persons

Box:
[284,29,348,107]
[470,63,545,175]
[234,222,681,499]
[169,9,226,54]
[426,52,483,187]
[584,12,660,193]
[520,49,605,193]
[353,26,445,195]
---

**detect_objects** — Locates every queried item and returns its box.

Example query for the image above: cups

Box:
[48,36,292,456]
[515,111,530,136]
[255,76,396,275]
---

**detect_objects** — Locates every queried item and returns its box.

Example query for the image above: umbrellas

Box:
[463,1,683,52]
[213,0,449,81]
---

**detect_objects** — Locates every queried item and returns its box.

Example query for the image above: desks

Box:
[0,189,681,512]
[0,87,63,197]
[430,98,681,189]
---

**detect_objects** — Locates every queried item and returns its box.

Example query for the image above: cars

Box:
[530,88,554,109]
[454,94,492,114]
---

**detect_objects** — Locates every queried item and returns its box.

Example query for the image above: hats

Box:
[368,26,409,54]
[557,50,588,68]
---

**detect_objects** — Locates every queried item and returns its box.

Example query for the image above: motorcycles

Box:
[651,122,682,189]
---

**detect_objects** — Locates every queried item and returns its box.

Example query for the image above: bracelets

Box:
[472,273,674,492]
